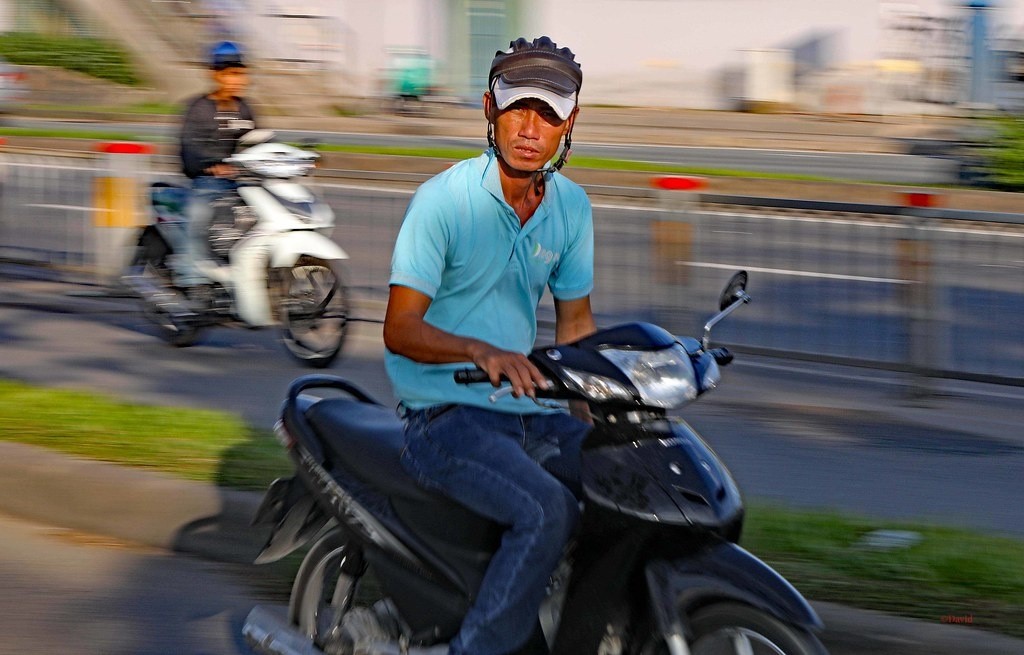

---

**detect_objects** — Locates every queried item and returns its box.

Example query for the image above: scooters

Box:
[117,139,349,369]
[243,270,823,655]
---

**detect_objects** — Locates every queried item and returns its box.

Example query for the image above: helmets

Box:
[207,40,250,72]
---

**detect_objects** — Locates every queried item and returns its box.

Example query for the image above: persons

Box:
[170,42,277,285]
[383,36,599,655]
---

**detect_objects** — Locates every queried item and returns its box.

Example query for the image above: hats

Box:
[487,36,581,119]
